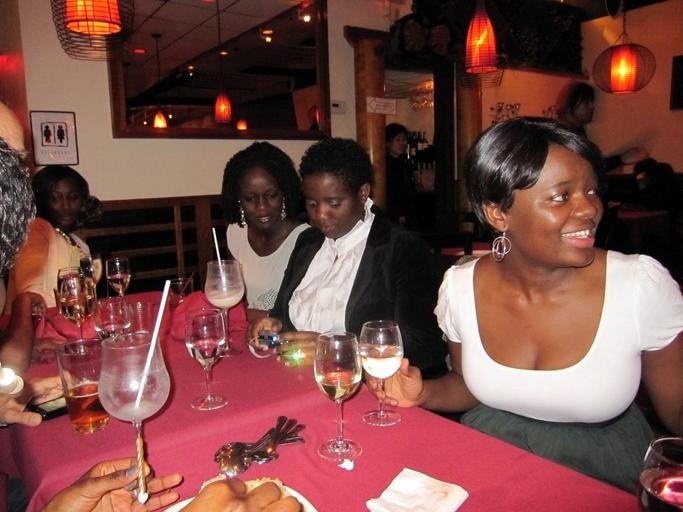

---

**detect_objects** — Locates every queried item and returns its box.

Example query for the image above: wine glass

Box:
[181,308,231,413]
[96,331,172,506]
[55,257,98,356]
[105,254,132,301]
[357,318,405,429]
[312,331,364,463]
[94,299,134,343]
[205,260,245,360]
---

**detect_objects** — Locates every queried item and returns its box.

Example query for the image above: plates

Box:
[162,485,318,512]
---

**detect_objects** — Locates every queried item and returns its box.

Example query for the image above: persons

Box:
[0,166,102,315]
[0,137,82,429]
[3,457,307,511]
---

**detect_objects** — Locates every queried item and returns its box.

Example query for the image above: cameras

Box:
[258,331,287,348]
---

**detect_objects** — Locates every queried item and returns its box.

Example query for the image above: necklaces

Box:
[56,227,72,246]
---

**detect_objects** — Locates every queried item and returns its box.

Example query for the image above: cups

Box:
[166,277,193,315]
[638,435,683,512]
[54,338,110,436]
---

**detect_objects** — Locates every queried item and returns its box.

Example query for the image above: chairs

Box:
[397,226,473,262]
[418,209,482,237]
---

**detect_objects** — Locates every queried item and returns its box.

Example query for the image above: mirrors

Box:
[102,0,334,142]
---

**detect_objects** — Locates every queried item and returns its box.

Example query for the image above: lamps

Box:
[51,0,134,64]
[148,32,167,129]
[591,2,656,96]
[211,1,233,124]
[235,116,249,130]
[463,0,495,76]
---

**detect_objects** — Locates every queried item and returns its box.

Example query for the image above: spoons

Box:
[214,412,306,477]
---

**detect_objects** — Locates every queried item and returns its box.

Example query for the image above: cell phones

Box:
[29,394,67,419]
